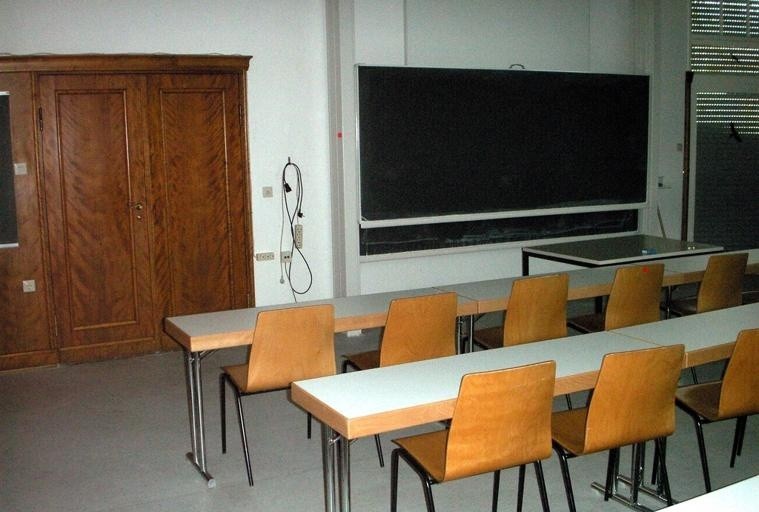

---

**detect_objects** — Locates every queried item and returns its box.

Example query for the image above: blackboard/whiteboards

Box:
[353,62,652,263]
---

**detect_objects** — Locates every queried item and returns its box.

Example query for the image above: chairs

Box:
[566,264,665,332]
[652,328,757,495]
[219,304,337,486]
[389,359,555,510]
[341,292,458,468]
[519,343,685,511]
[669,252,750,317]
[462,272,571,413]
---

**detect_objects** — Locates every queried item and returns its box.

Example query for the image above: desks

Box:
[519,232,729,277]
[608,304,758,505]
[648,249,757,319]
[291,330,685,511]
[433,259,685,353]
[165,287,477,488]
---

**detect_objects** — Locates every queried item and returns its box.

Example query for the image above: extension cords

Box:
[295,225,302,249]
